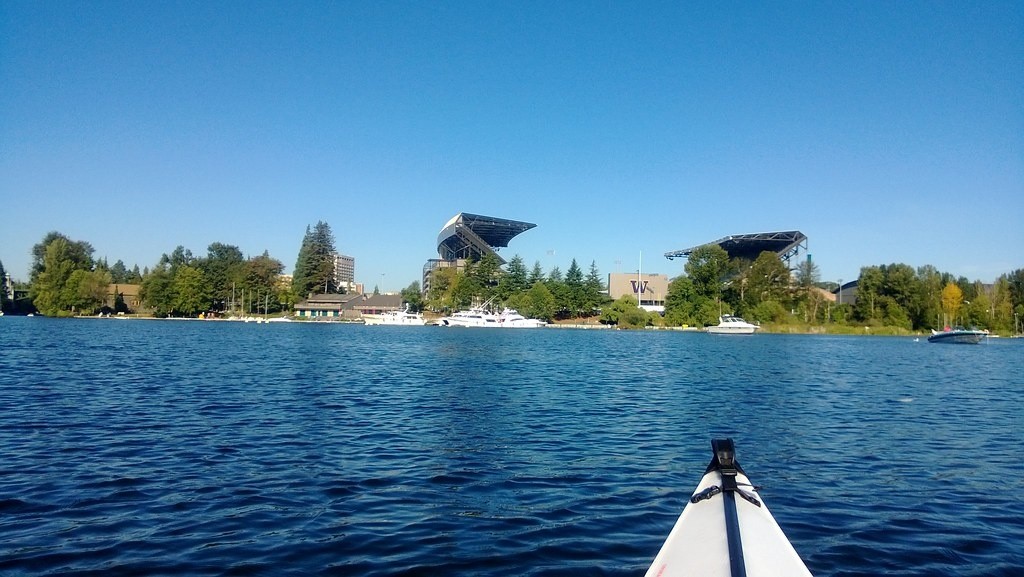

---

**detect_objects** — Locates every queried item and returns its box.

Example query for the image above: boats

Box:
[425,294,548,328]
[927,326,990,345]
[707,316,760,334]
[361,311,428,326]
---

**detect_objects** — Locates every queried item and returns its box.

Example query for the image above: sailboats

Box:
[205,283,292,323]
